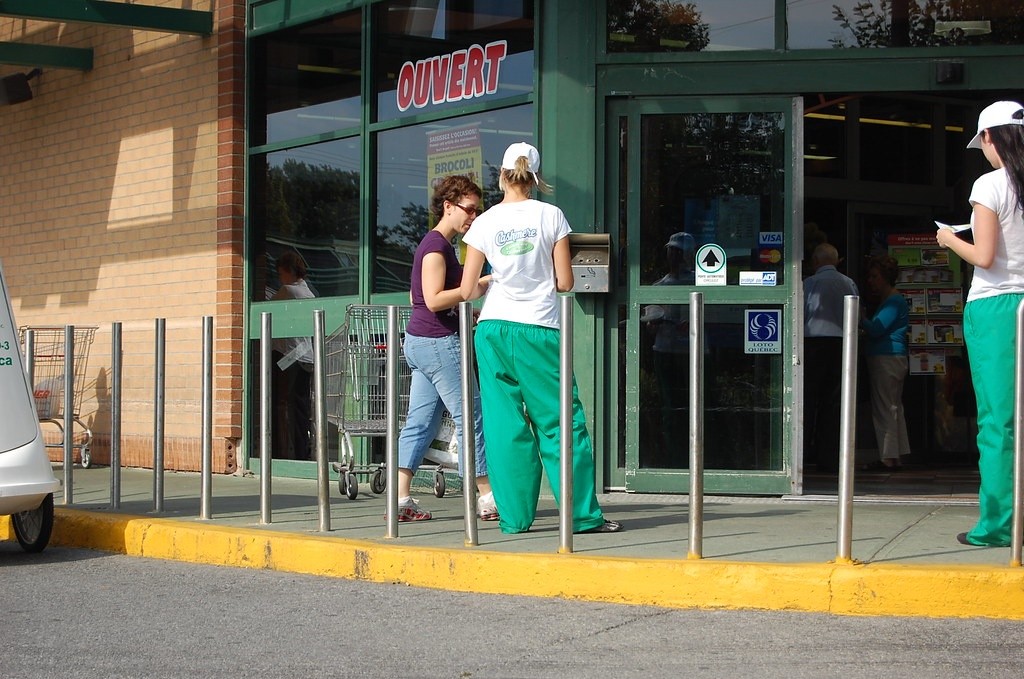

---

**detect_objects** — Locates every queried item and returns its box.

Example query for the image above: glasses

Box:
[447,199,482,217]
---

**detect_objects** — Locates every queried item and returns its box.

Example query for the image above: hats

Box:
[966,101,1024,149]
[502,142,540,185]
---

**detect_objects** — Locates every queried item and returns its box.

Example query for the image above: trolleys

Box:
[15,325,98,470]
[307,304,455,501]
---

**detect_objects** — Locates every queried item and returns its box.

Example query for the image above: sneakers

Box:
[478,495,500,521]
[383,497,432,521]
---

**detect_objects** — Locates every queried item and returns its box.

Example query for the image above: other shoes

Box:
[868,461,906,475]
[816,460,834,473]
[957,532,985,546]
[585,519,623,532]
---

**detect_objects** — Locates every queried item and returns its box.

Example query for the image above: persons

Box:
[461,141,625,534]
[804,243,860,473]
[936,100,1024,548]
[646,232,722,470]
[269,250,317,460]
[860,256,911,472]
[383,175,500,524]
